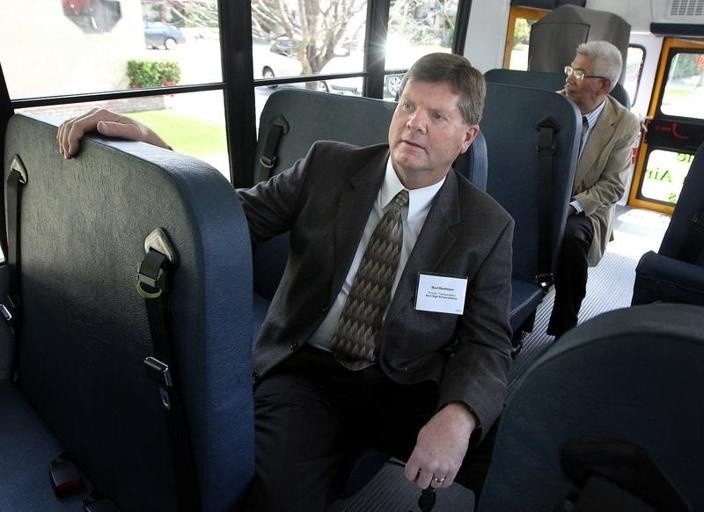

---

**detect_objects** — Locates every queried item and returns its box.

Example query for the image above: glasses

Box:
[563,65,608,82]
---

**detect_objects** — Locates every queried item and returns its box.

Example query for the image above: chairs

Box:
[0,65,704,512]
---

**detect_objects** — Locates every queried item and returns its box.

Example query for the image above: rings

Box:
[434,477,445,483]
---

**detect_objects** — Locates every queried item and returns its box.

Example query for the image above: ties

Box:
[328,189,409,372]
[577,117,589,157]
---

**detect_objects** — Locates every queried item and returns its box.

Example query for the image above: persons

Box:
[58,52,516,512]
[511,40,642,358]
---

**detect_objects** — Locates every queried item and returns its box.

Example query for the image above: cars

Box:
[385,45,440,98]
[260,35,364,95]
[143,20,186,50]
